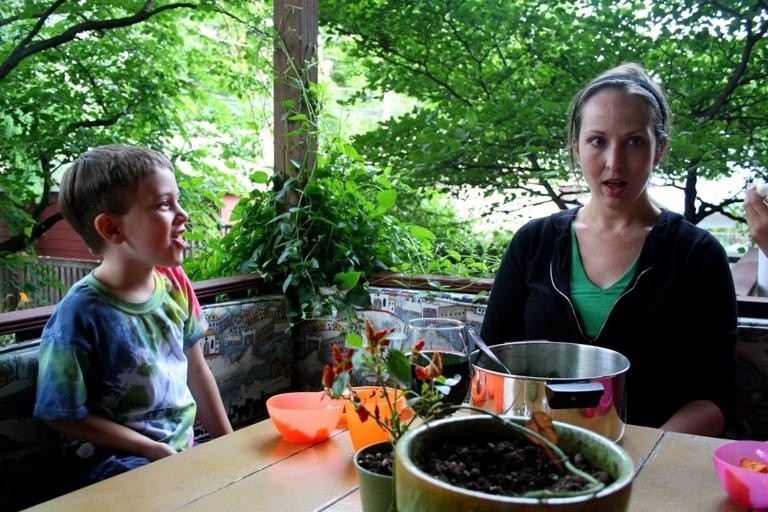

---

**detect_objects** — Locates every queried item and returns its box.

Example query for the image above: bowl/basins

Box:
[264,389,340,443]
[713,440,768,510]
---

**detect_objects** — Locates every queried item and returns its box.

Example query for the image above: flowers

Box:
[321,319,441,463]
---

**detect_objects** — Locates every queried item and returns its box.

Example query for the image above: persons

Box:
[33,143,234,487]
[475,62,736,437]
[730,180,767,299]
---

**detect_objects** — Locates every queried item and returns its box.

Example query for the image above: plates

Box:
[339,386,408,426]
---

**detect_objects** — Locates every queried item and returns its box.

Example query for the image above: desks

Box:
[20,391,768,512]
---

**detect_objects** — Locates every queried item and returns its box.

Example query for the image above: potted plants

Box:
[331,331,635,512]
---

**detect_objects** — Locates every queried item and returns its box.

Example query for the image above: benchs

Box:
[0,287,768,512]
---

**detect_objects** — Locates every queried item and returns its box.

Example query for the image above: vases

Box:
[353,441,397,512]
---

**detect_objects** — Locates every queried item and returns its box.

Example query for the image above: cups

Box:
[348,385,411,454]
[398,316,468,418]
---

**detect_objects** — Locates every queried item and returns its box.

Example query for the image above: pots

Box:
[469,340,633,446]
